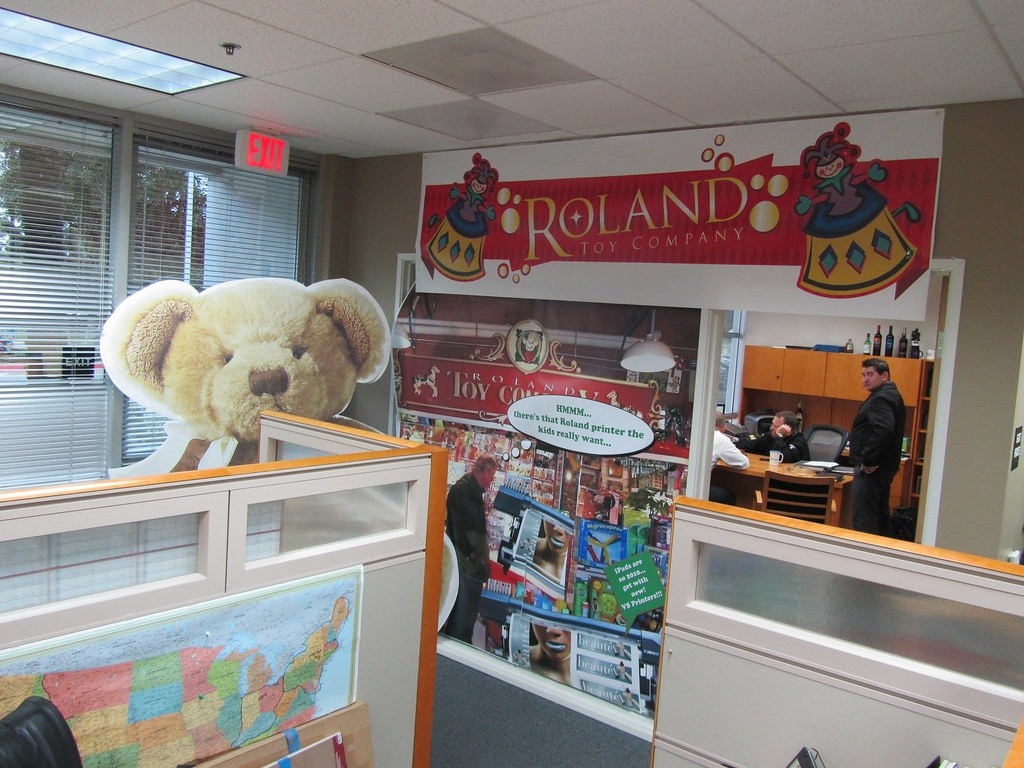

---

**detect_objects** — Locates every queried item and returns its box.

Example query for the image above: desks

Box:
[716,452,853,528]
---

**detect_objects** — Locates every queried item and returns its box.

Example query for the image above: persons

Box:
[529,623,571,685]
[709,411,749,506]
[446,454,498,643]
[533,520,570,580]
[848,358,907,536]
[732,411,811,463]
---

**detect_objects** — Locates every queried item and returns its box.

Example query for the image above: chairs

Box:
[753,470,835,525]
[803,422,851,462]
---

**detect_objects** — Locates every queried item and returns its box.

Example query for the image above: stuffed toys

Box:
[101,277,453,621]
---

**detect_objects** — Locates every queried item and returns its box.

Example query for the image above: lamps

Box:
[620,308,676,372]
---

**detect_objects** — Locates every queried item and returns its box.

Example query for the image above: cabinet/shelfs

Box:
[743,345,934,512]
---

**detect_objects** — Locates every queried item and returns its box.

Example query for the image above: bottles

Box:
[885,326,894,356]
[846,338,853,353]
[795,399,803,434]
[873,324,882,356]
[863,333,871,355]
[899,327,908,358]
[911,328,920,349]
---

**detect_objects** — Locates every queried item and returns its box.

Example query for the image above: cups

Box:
[770,450,784,467]
[911,348,923,359]
